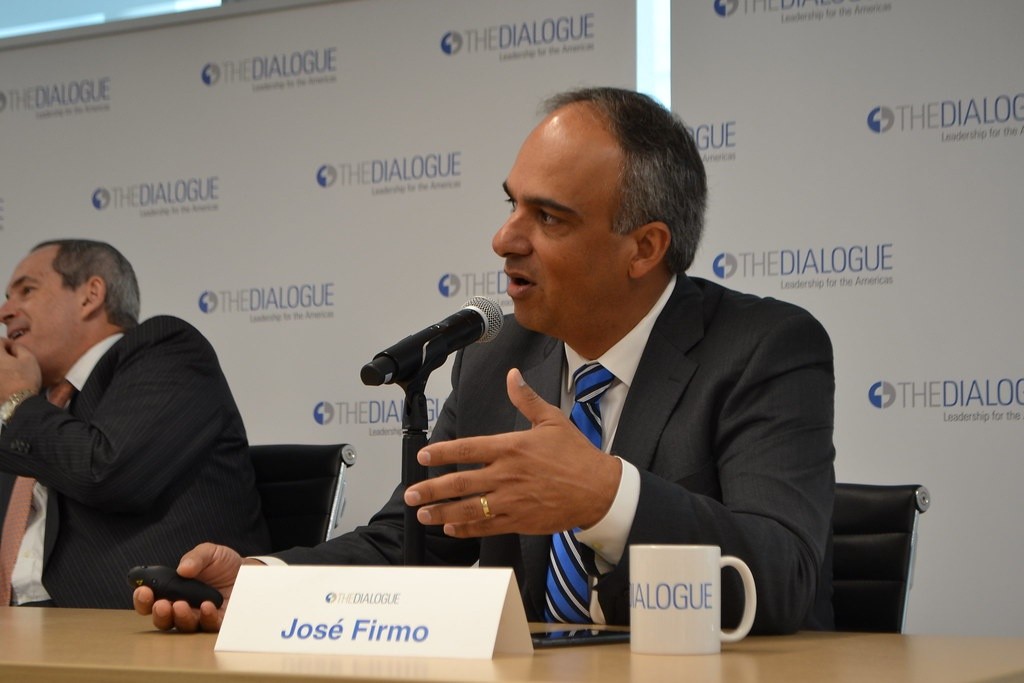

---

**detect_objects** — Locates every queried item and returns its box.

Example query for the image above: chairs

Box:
[824,481,929,634]
[250,441,355,557]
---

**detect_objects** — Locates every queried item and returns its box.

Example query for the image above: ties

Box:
[543,362,616,639]
[0,381,75,606]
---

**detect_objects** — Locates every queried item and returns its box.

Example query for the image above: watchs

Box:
[0,389,34,427]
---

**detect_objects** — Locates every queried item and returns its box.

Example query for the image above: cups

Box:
[629,545,757,656]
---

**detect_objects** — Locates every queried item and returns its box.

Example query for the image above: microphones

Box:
[361,296,504,386]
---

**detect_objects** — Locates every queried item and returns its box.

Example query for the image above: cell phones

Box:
[530,627,630,648]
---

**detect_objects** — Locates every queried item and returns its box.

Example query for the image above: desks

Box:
[1,608,1024,683]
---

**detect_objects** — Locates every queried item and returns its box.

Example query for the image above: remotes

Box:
[126,565,224,611]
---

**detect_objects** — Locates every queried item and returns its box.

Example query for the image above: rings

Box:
[480,495,491,520]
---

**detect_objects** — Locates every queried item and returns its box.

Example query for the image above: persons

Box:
[133,88,837,634]
[0,239,272,610]
